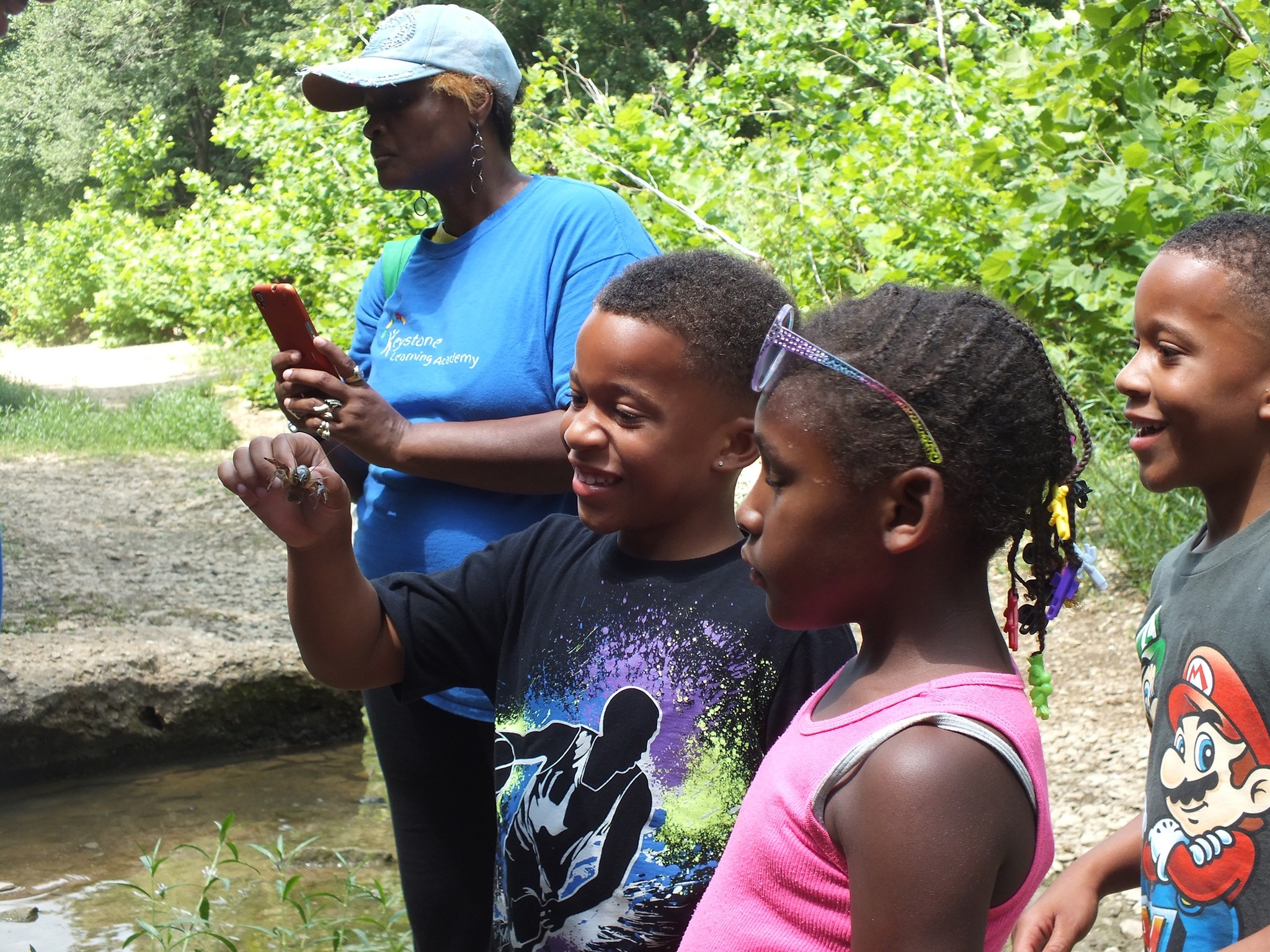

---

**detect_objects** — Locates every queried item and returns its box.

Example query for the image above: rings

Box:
[287,394,306,433]
[313,396,343,421]
[313,421,330,441]
[345,365,364,384]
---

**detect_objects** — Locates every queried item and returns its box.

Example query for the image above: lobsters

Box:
[263,457,329,510]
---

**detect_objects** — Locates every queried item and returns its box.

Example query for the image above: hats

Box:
[302,4,524,113]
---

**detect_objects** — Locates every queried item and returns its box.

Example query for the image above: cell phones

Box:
[252,283,342,393]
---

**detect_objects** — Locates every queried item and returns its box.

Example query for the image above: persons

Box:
[266,6,662,952]
[675,282,1094,952]
[1011,209,1270,952]
[218,248,859,952]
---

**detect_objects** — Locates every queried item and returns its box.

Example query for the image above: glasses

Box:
[751,304,945,466]
